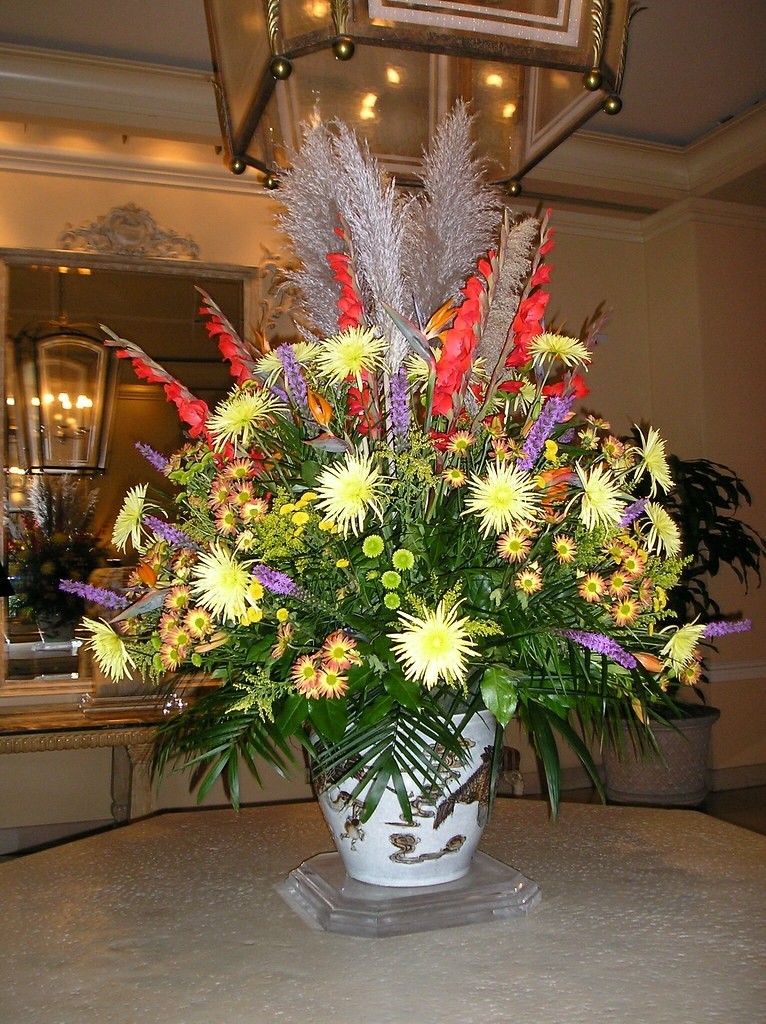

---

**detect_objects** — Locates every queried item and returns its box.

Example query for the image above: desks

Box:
[1,800,766,1024]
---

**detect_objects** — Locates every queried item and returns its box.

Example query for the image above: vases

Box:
[35,618,77,641]
[307,707,497,887]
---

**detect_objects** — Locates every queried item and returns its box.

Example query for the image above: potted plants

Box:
[609,421,766,807]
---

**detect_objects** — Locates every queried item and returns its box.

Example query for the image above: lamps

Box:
[6,271,119,479]
[203,1,648,198]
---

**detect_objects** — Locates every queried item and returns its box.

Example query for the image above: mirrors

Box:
[0,202,263,735]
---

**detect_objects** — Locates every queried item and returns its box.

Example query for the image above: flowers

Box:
[1,472,114,625]
[56,90,751,828]
